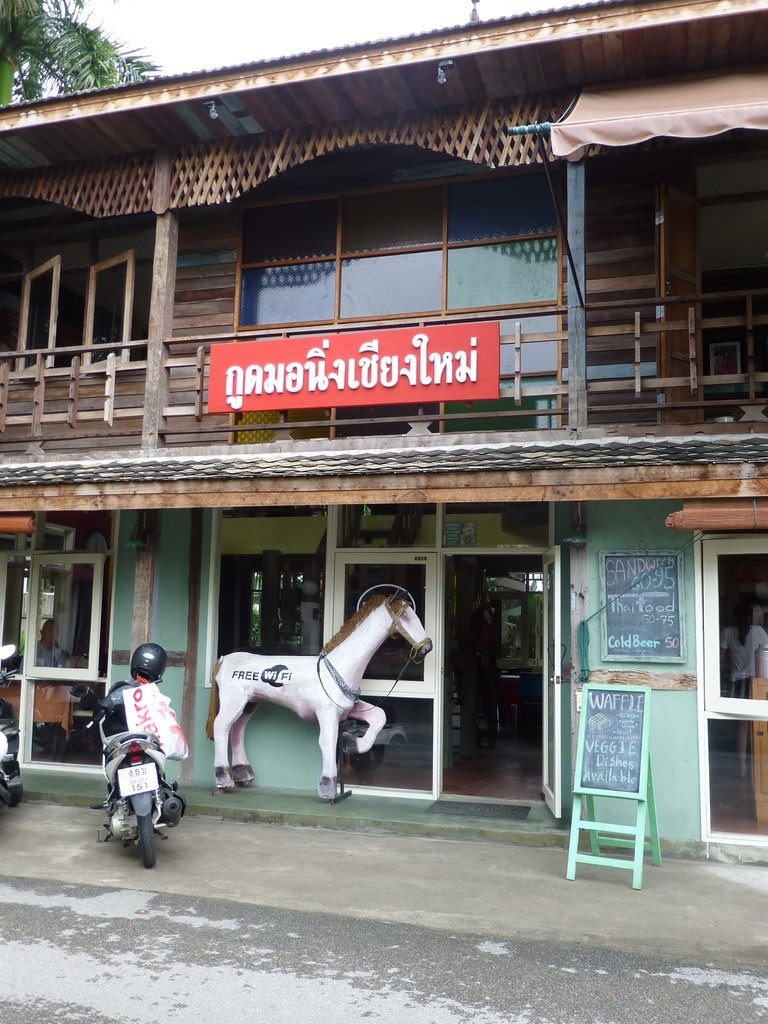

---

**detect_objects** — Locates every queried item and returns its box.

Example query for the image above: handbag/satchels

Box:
[122,684,189,761]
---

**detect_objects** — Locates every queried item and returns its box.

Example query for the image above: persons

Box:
[719,602,768,779]
[18,619,69,683]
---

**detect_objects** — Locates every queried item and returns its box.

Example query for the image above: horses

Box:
[204,586,434,800]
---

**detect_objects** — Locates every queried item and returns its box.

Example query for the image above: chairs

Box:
[514,673,544,734]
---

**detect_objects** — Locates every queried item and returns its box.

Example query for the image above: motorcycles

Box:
[68,643,187,869]
[0,644,26,813]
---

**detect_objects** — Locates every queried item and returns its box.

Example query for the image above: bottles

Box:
[754,643,768,678]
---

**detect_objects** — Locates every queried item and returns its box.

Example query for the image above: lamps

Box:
[437,60,455,85]
[201,99,219,120]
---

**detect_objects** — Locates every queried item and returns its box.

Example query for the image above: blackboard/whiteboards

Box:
[573,682,653,802]
[597,549,690,665]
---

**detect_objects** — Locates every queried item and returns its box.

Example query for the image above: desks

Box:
[0,685,74,762]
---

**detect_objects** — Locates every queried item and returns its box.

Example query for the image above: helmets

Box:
[131,643,166,680]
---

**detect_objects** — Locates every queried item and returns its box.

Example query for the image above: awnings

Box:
[548,74,768,163]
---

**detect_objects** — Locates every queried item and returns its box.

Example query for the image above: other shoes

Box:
[740,766,747,779]
